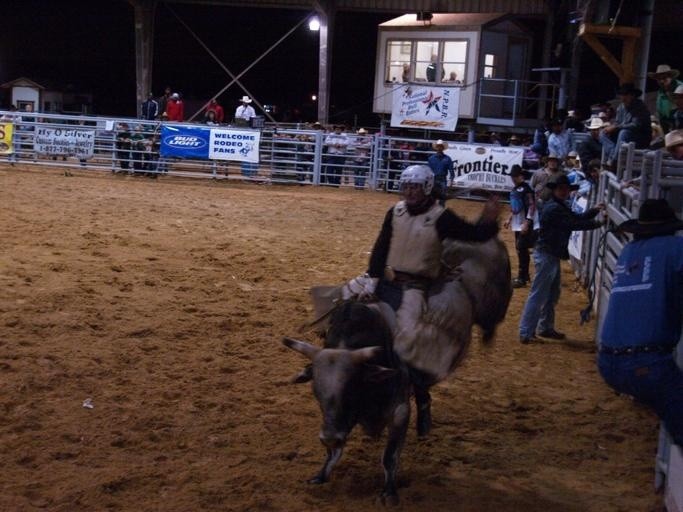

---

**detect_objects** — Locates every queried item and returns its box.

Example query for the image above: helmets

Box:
[395,163,436,195]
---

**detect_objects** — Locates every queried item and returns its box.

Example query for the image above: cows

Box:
[280,188,514,512]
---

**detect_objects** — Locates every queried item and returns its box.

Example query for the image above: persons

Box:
[400,63,412,82]
[1,104,89,166]
[269,105,421,193]
[115,86,263,179]
[528,65,683,245]
[518,175,609,346]
[596,199,683,458]
[352,163,506,345]
[502,165,538,287]
[421,129,535,208]
[425,55,444,82]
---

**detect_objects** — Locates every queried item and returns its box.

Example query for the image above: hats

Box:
[646,64,681,82]
[356,127,369,135]
[239,95,253,104]
[505,164,529,176]
[664,128,683,152]
[614,82,642,98]
[615,198,683,237]
[672,84,683,95]
[545,174,580,191]
[582,111,611,130]
[310,121,323,128]
[540,151,562,160]
[649,113,662,135]
[332,122,346,130]
[431,138,448,151]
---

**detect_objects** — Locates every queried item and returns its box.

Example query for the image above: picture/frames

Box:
[400,42,410,55]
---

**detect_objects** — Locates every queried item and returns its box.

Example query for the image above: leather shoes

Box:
[294,366,316,384]
[415,391,432,437]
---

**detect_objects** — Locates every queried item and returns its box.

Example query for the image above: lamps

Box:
[416,9,433,26]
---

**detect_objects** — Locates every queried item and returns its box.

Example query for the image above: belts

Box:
[599,344,670,357]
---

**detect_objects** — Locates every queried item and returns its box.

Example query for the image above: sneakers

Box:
[117,170,157,178]
[519,335,545,345]
[538,329,565,339]
[511,276,530,288]
